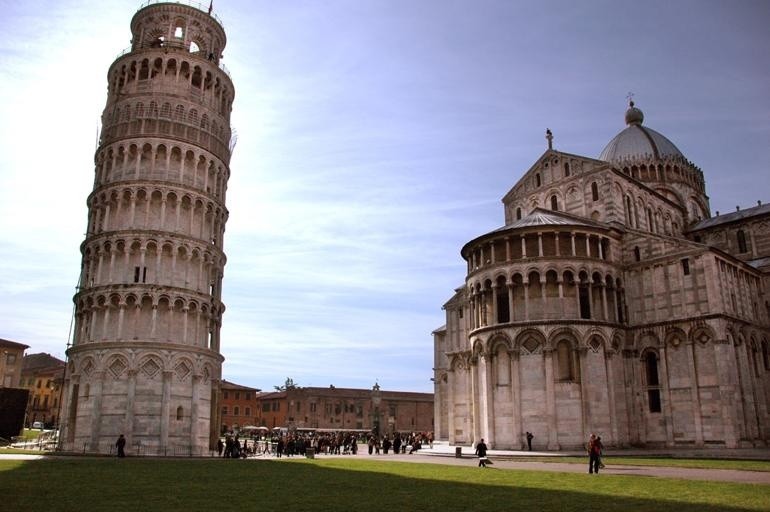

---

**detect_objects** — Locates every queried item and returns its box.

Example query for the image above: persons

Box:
[587,434,605,474]
[115,434,126,458]
[526,431,534,451]
[476,438,487,468]
[217,426,433,459]
